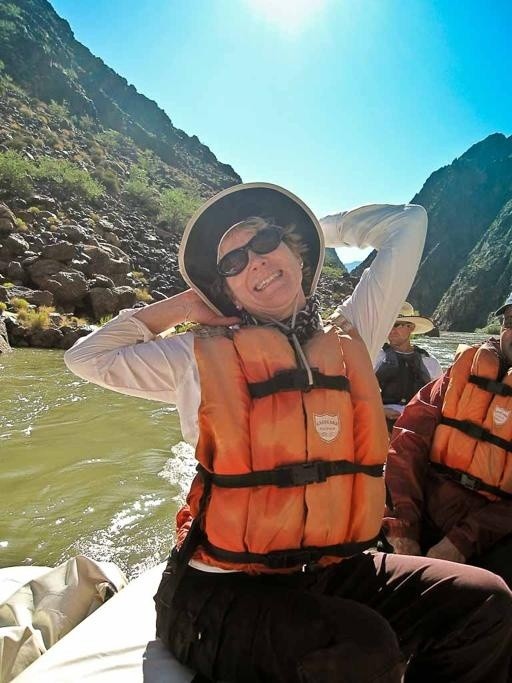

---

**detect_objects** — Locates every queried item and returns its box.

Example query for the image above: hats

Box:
[178,182,325,317]
[397,302,434,334]
[494,292,512,316]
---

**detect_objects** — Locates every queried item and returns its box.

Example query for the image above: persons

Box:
[377,293,512,584]
[372,300,443,436]
[62,182,511,679]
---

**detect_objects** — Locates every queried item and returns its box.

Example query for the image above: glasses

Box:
[394,323,410,328]
[217,229,281,277]
